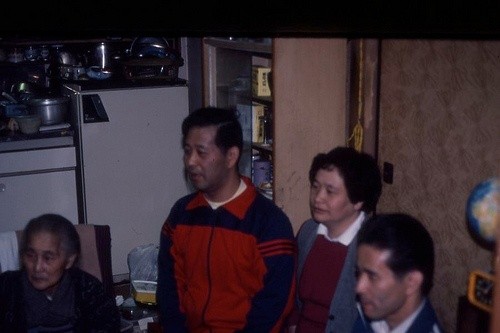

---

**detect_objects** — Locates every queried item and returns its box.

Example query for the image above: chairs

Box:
[16,223,114,300]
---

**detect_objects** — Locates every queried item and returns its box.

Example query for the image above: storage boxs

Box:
[236,103,264,142]
[251,64,271,95]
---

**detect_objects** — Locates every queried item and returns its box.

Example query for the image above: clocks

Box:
[468,270,494,314]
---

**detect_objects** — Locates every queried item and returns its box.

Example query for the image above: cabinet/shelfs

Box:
[203,35,351,239]
[0,146,80,232]
[456,296,490,332]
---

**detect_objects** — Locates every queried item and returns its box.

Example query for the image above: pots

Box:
[26,93,71,130]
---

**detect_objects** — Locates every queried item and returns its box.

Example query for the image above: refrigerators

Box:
[76,86,190,285]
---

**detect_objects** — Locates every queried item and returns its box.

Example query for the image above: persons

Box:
[154,107,295,333]
[0,214,120,333]
[295,147,382,333]
[355,212,444,333]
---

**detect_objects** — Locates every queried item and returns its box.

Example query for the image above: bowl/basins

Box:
[14,115,41,135]
